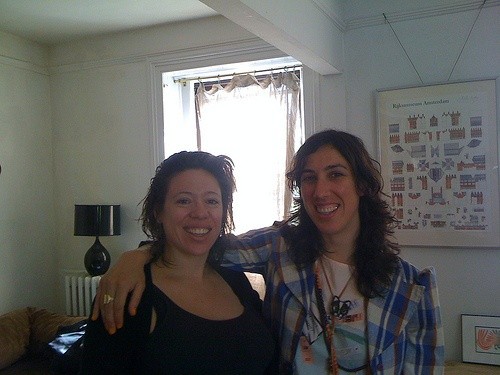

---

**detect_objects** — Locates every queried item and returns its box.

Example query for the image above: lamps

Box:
[73,204,121,276]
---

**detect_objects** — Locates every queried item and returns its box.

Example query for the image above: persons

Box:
[78,151,282,375]
[92,128,447,375]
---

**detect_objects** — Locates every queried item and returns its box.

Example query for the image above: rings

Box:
[102,293,114,305]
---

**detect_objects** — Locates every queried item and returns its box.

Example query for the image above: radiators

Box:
[62,267,104,317]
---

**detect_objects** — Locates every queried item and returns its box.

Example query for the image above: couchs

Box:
[0,306,88,375]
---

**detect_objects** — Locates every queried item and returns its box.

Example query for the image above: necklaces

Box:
[314,250,370,375]
